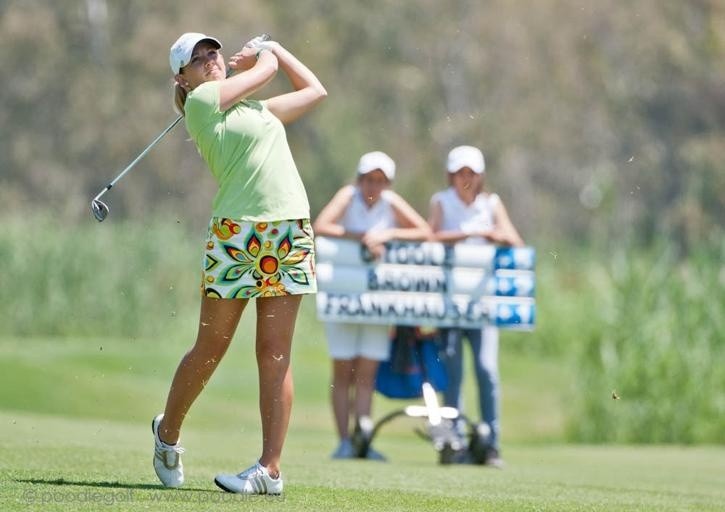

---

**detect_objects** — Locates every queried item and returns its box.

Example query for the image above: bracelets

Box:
[255,48,265,60]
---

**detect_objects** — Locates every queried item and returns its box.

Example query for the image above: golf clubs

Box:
[92,32,272,223]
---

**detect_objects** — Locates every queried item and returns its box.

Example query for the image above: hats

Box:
[357,150,396,185]
[446,145,487,176]
[168,31,223,75]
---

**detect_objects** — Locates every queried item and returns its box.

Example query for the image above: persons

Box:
[425,145,526,467]
[151,32,328,496]
[311,152,433,461]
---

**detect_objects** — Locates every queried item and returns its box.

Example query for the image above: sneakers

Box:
[149,412,185,489]
[213,460,285,496]
